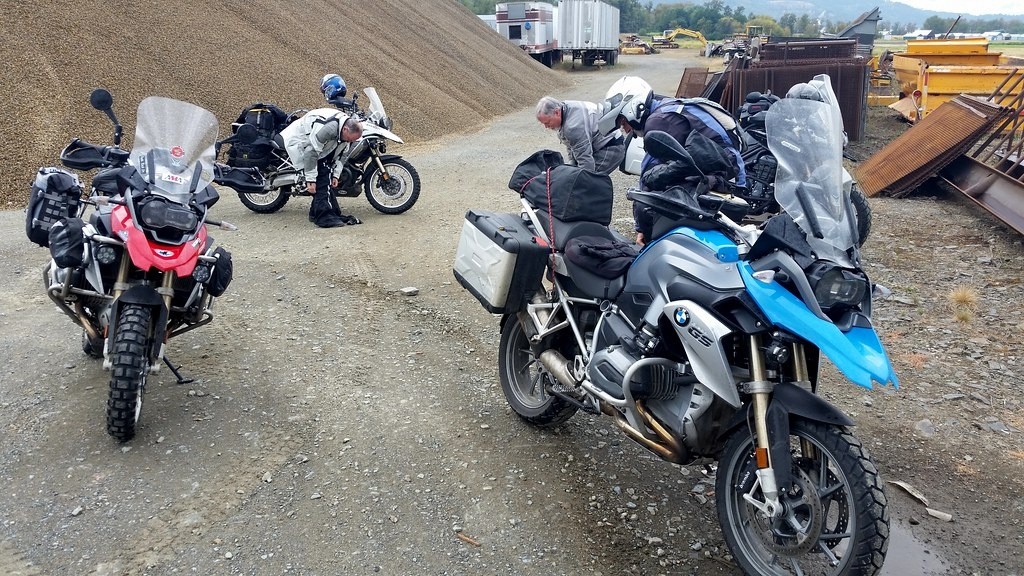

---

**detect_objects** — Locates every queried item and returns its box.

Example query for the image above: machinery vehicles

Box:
[619,26,770,57]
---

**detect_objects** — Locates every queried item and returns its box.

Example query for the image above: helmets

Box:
[320,73,346,101]
[596,75,654,137]
[784,84,820,115]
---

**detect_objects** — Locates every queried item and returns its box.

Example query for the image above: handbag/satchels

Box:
[509,149,613,225]
[49,215,83,269]
[26,169,81,247]
[245,106,274,137]
[205,247,233,298]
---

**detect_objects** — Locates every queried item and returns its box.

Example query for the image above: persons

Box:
[536,95,625,176]
[279,108,363,228]
[706,42,714,56]
[597,75,747,246]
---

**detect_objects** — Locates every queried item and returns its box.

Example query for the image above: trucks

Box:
[476,0,620,69]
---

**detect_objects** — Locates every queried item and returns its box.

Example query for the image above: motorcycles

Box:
[498,89,890,576]
[43,88,238,440]
[212,73,421,215]
